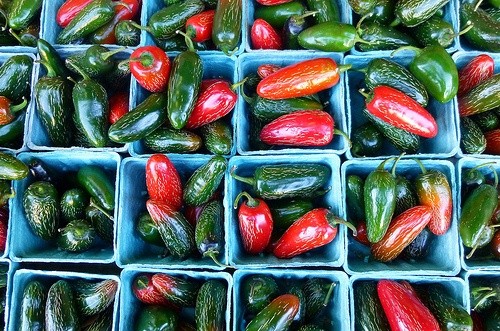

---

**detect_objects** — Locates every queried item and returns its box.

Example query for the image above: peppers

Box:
[0,0,500,331]
